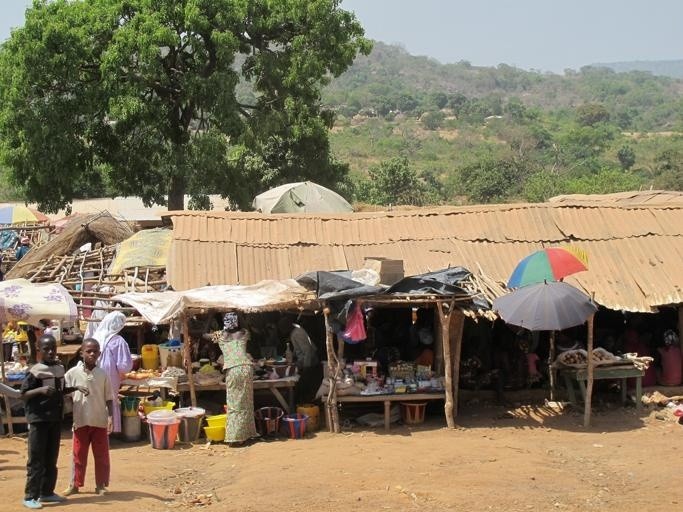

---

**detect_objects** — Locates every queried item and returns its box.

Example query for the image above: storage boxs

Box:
[363,258,403,286]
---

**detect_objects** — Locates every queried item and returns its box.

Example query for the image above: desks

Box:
[108,374,173,400]
[174,374,302,415]
[560,365,645,420]
[0,367,71,437]
[325,374,450,433]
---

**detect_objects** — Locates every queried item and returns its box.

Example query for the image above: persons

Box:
[203,311,260,447]
[19,333,91,510]
[0,381,23,401]
[92,310,134,439]
[188,310,683,394]
[62,337,114,497]
[278,319,324,409]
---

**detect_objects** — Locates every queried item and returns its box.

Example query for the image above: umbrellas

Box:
[0,204,49,225]
[505,246,588,289]
[491,278,600,334]
[252,179,355,214]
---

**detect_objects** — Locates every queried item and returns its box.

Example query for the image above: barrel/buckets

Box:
[280,412,309,440]
[142,401,177,416]
[141,343,159,370]
[400,401,428,425]
[254,406,285,436]
[173,407,206,442]
[297,402,321,432]
[147,419,181,450]
[147,410,176,422]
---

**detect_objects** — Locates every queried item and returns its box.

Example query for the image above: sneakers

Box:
[95,487,107,494]
[62,487,78,496]
[24,500,42,508]
[38,495,66,502]
